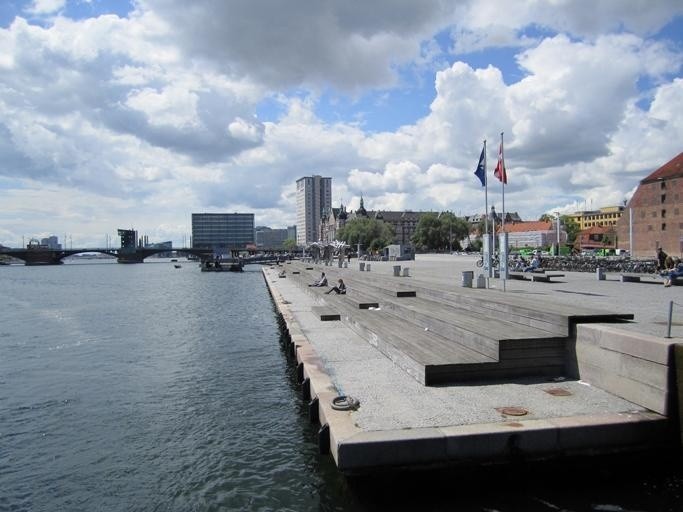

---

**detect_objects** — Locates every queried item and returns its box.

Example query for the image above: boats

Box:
[201,257,245,272]
[0,258,10,265]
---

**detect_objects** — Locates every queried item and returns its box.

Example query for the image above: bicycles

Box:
[476,254,658,274]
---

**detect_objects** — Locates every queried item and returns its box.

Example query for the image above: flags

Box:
[494,143,507,184]
[474,147,485,186]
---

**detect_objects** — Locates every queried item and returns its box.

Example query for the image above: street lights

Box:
[489,206,498,254]
[553,211,559,243]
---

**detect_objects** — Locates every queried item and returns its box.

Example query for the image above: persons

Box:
[308,272,327,287]
[324,279,346,294]
[657,247,668,270]
[655,256,683,287]
[490,206,502,226]
[523,254,542,272]
